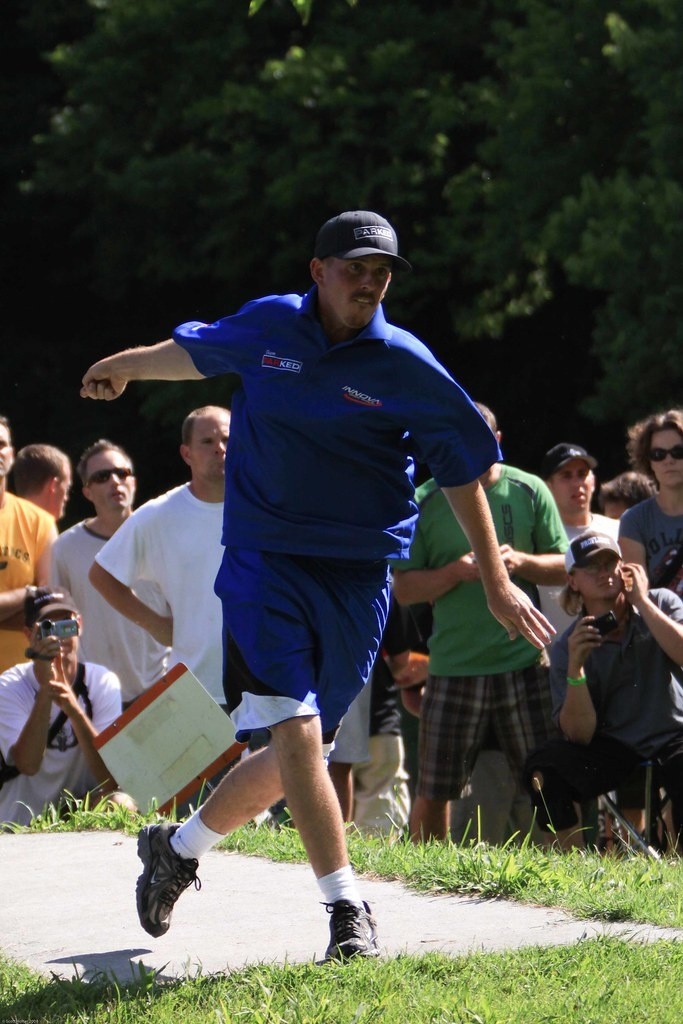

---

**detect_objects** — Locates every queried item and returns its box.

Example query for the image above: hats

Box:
[24,585,79,625]
[565,531,623,574]
[543,443,598,480]
[315,210,411,284]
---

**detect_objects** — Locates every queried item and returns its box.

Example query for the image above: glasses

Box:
[585,561,620,574]
[86,468,133,487]
[650,445,683,461]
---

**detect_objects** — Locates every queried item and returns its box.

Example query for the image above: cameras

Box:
[38,619,79,639]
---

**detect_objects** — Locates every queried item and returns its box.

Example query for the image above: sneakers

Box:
[320,900,380,960]
[135,823,201,938]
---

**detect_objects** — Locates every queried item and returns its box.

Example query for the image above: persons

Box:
[0,416,58,675]
[48,438,171,711]
[87,406,272,799]
[80,211,557,961]
[387,398,571,845]
[326,602,433,840]
[599,471,656,520]
[619,411,683,850]
[524,531,683,856]
[0,584,123,827]
[541,442,621,647]
[13,444,72,521]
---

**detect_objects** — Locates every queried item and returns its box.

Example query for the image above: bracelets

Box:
[567,675,586,686]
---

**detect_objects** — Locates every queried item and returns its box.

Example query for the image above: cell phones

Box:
[586,610,618,637]
